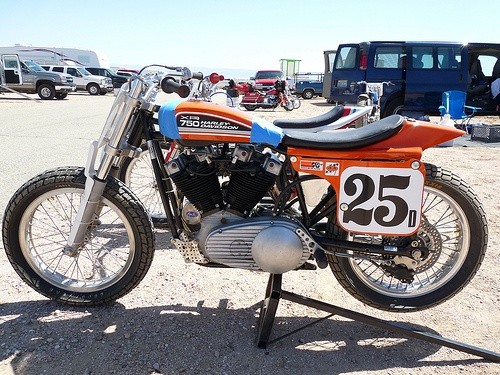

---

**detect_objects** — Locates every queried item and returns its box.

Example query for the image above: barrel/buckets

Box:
[436,114,454,147]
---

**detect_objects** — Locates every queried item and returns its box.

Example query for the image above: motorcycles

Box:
[117,72,373,232]
[239,80,295,112]
[1,63,489,363]
[284,76,301,109]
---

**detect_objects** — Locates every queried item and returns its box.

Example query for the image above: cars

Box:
[116,68,139,78]
[250,69,292,96]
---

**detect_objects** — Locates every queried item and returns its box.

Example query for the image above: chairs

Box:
[457,59,490,95]
[438,90,482,135]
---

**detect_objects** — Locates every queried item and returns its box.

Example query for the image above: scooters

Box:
[353,79,396,123]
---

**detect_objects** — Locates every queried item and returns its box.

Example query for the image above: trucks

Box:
[295,73,325,99]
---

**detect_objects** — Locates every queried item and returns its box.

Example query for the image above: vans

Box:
[330,41,500,120]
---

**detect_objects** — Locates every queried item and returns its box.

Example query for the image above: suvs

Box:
[84,68,131,88]
[0,54,77,100]
[38,65,114,95]
